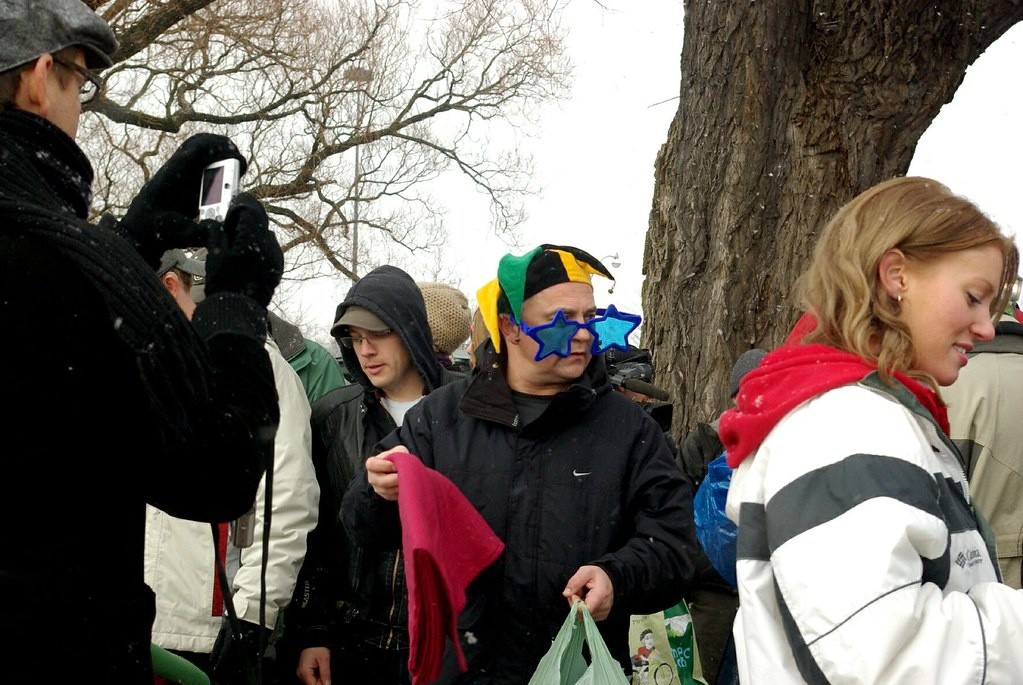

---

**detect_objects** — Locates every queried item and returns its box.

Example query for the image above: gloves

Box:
[196,192,283,310]
[120,132,248,248]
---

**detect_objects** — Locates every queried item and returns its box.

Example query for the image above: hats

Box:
[330,304,392,338]
[729,349,770,397]
[415,281,472,355]
[0,0,121,73]
[155,247,209,303]
[475,243,616,369]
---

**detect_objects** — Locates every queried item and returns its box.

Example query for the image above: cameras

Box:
[199,159,239,222]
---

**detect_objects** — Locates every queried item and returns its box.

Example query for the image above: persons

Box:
[0,2,287,685]
[147,175,1023,685]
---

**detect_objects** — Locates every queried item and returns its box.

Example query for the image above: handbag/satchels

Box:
[208,614,291,685]
[528,599,630,685]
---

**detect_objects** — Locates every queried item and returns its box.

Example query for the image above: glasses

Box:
[340,328,397,348]
[52,52,104,105]
[509,303,642,362]
